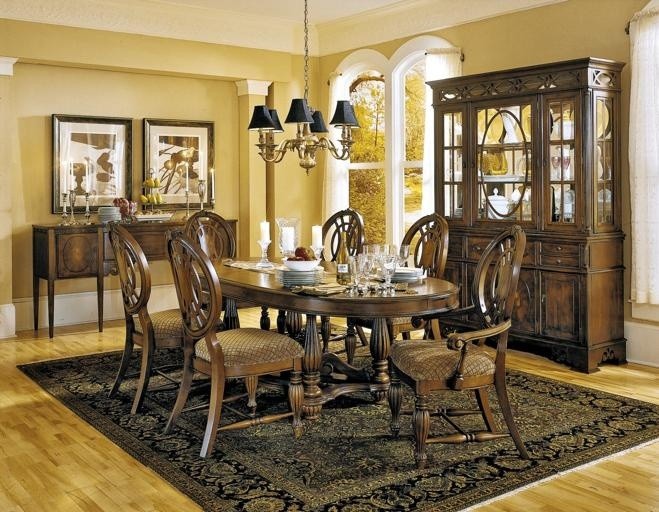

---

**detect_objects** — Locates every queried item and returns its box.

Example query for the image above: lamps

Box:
[247,1,362,177]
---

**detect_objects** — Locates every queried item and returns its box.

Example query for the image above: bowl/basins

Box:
[281,257,322,270]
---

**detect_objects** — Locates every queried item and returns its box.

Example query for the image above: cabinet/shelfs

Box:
[425,56,631,374]
[32,219,239,338]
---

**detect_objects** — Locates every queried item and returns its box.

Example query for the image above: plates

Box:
[515,106,553,142]
[478,110,503,141]
[98,207,122,224]
[596,101,610,137]
[376,266,423,283]
[278,266,324,288]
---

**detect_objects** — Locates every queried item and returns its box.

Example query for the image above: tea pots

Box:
[517,151,532,182]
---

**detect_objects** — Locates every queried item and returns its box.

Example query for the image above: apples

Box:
[288,245,316,260]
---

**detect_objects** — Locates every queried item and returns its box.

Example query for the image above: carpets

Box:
[16,322,658,512]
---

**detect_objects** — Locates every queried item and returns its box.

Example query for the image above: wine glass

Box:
[552,149,570,181]
[347,244,411,296]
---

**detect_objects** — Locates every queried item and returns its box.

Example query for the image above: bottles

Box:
[488,151,509,175]
[486,184,508,221]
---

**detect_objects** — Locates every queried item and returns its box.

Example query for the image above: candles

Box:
[63,161,66,194]
[70,159,74,191]
[199,151,203,181]
[63,142,215,200]
[185,162,188,193]
[85,160,89,194]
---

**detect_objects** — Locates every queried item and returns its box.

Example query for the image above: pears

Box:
[139,193,162,203]
[143,177,160,187]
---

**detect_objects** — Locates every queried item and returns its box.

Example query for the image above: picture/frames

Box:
[51,113,133,215]
[142,118,215,211]
